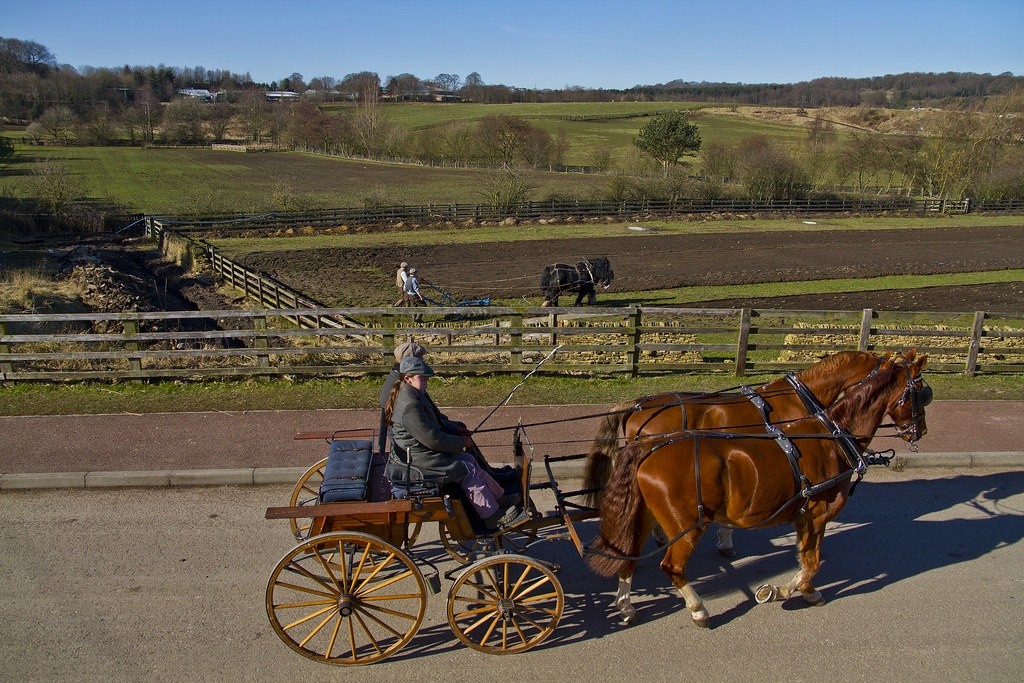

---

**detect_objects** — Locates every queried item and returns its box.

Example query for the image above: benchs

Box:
[316,427,449,502]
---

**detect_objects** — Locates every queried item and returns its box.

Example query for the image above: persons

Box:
[394,262,411,308]
[379,342,522,496]
[403,268,427,323]
[385,357,522,532]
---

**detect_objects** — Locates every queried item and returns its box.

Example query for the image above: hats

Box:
[401,262,408,268]
[410,268,418,274]
[400,357,434,377]
[394,344,426,362]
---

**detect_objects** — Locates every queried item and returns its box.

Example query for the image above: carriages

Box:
[419,255,614,321]
[263,343,934,667]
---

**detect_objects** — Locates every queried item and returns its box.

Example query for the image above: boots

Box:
[405,303,410,308]
[393,299,403,307]
[414,314,424,323]
[411,313,416,322]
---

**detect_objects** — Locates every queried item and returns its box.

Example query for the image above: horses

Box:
[539,255,615,308]
[575,347,930,629]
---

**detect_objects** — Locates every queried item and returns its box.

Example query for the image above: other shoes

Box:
[499,494,522,506]
[494,469,519,489]
[491,465,511,473]
[484,505,518,529]
